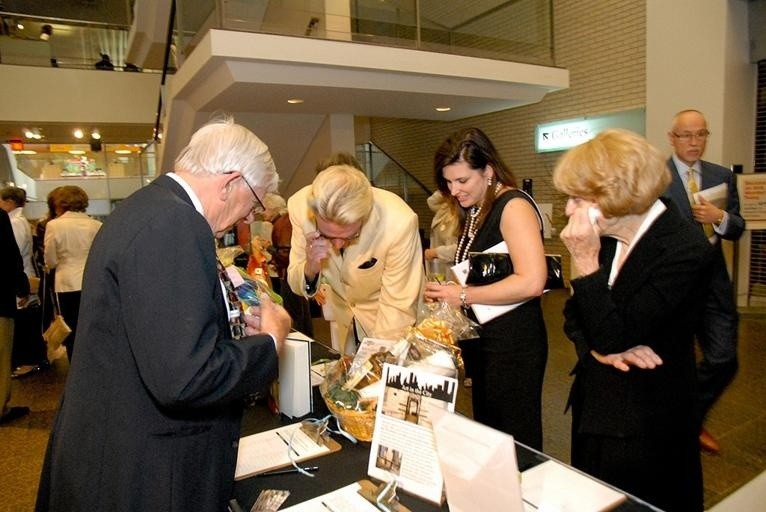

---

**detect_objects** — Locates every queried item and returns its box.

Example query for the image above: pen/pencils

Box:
[259,466,319,476]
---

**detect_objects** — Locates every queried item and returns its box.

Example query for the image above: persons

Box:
[284,165,423,360]
[423,128,548,452]
[33,108,294,512]
[658,110,745,453]
[0,186,104,422]
[315,149,365,356]
[424,190,472,283]
[261,193,313,339]
[552,127,703,512]
[458,339,472,388]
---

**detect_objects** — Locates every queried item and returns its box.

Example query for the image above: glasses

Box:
[315,217,361,240]
[223,171,266,216]
[673,129,710,141]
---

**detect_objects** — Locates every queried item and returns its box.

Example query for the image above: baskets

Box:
[319,355,377,443]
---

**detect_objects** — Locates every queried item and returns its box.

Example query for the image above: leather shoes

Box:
[699,429,721,454]
[9,364,40,378]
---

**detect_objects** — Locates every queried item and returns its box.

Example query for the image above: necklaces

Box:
[455,181,502,266]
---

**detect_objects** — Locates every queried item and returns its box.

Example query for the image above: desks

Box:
[234,327,663,512]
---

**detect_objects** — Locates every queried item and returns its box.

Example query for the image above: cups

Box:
[424,257,447,283]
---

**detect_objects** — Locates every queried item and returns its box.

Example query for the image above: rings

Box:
[308,241,313,250]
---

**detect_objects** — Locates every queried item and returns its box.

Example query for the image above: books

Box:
[693,182,729,246]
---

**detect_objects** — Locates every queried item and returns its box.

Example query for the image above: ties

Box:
[687,168,715,240]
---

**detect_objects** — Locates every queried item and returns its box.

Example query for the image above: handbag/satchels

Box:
[465,251,565,290]
[42,314,73,351]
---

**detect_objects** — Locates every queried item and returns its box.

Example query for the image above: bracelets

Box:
[717,210,723,224]
[461,285,470,310]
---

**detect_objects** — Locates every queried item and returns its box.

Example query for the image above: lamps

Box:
[39,25,52,40]
[91,144,101,151]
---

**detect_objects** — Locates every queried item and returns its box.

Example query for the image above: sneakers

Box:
[0,406,31,426]
